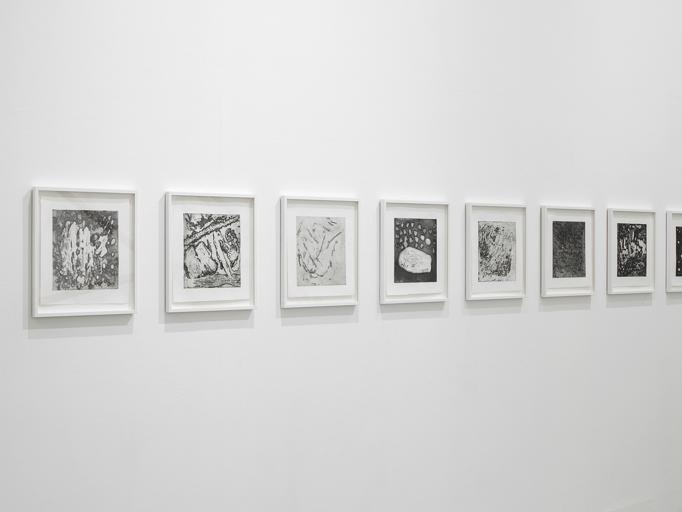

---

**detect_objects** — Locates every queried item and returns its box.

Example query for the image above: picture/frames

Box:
[664,209,682,295]
[375,198,453,306]
[29,184,141,319]
[539,205,597,299]
[605,207,658,296]
[162,190,260,314]
[463,201,528,302]
[277,193,361,310]
